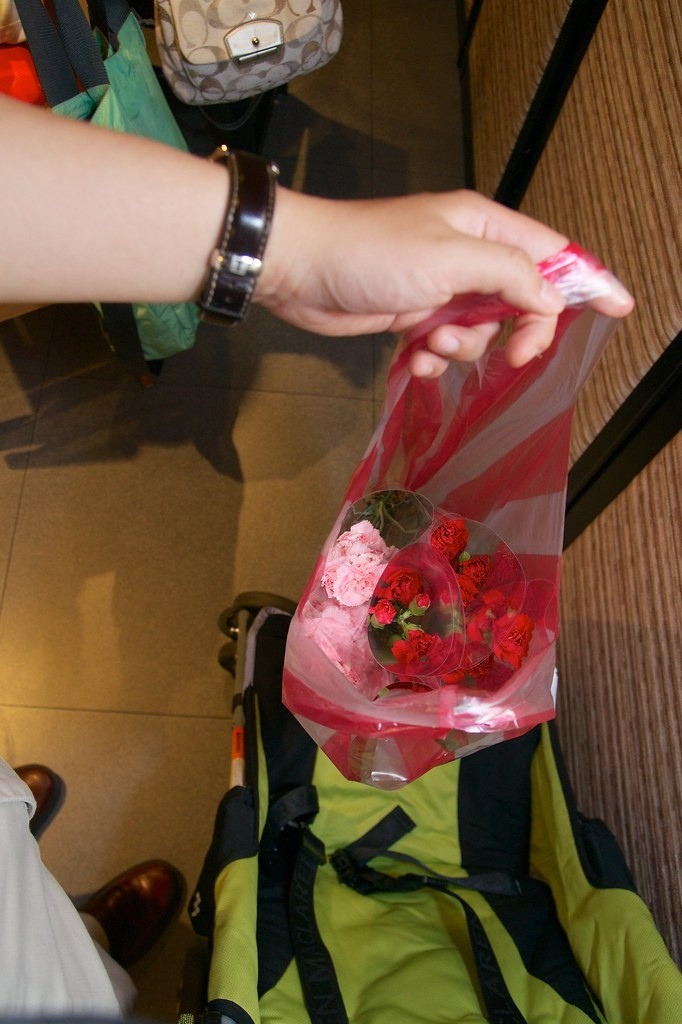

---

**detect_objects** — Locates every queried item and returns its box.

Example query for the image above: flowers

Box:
[303,480,537,703]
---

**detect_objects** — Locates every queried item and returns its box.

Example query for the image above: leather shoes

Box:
[9,763,55,835]
[76,860,183,971]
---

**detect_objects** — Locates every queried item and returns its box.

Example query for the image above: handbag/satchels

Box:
[13,0,209,362]
[155,0,343,105]
[281,246,629,791]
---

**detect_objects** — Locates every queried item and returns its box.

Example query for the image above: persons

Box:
[0,94,636,1024]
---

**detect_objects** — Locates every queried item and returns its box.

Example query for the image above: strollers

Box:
[182,583,682,1024]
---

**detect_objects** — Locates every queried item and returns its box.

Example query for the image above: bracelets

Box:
[200,146,278,326]
